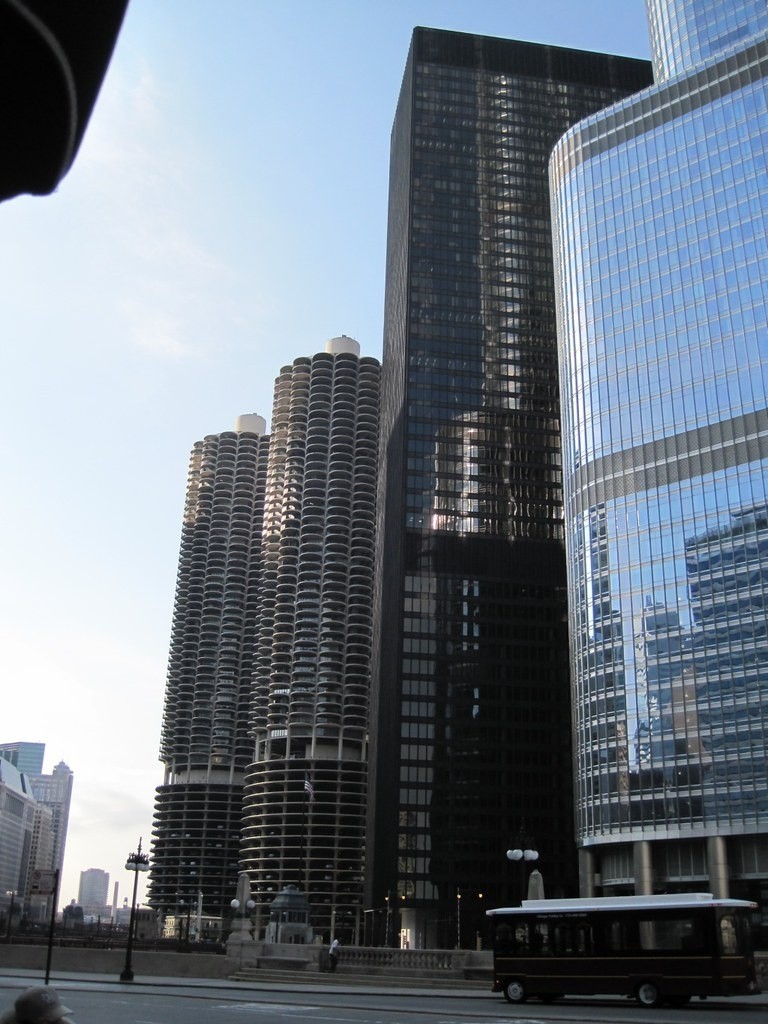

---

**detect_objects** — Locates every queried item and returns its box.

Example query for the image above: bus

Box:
[485,891,763,1011]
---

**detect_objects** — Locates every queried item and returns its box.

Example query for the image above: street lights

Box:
[118,835,151,981]
[504,815,541,908]
